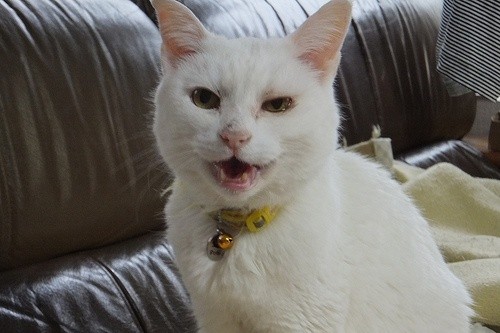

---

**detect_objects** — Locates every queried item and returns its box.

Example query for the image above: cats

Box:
[149,1,477,333]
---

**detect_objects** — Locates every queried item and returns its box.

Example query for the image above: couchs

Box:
[0,0,500,333]
